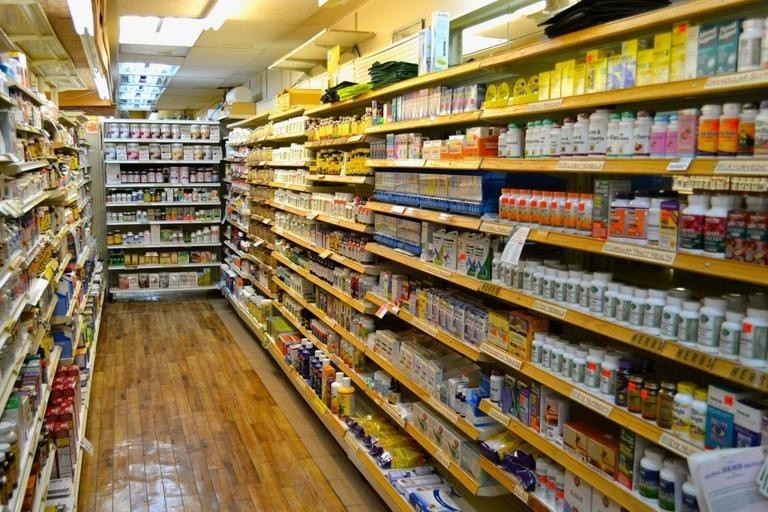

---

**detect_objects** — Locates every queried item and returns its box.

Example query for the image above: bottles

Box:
[697,100,768,156]
[498,187,594,232]
[671,378,707,446]
[455,383,466,418]
[529,330,633,395]
[633,447,702,511]
[498,105,678,158]
[106,211,148,222]
[111,189,145,201]
[332,267,378,306]
[191,227,211,244]
[31,418,51,479]
[297,337,368,420]
[489,366,505,402]
[121,231,144,245]
[327,225,376,266]
[681,192,762,257]
[491,252,768,370]
[310,192,354,218]
[0,421,21,506]
[608,187,678,244]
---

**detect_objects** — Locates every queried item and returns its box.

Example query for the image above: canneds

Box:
[640,381,655,418]
[0,443,18,505]
[657,381,675,429]
[626,374,645,414]
